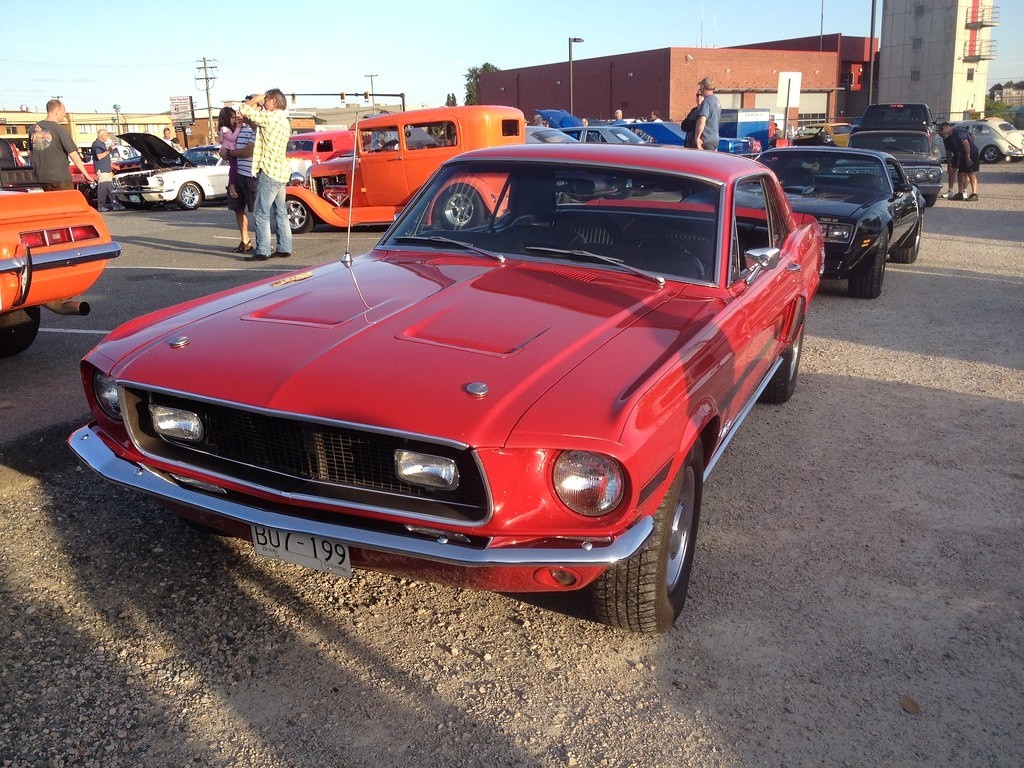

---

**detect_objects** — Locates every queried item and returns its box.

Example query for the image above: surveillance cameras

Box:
[688,55,694,61]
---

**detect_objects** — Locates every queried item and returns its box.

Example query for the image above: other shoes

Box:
[233,240,253,253]
[965,195,978,201]
[229,187,238,198]
[949,193,963,200]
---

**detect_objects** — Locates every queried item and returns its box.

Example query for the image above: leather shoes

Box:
[244,254,270,261]
[270,251,291,257]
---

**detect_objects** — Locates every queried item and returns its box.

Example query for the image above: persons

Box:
[935,122,980,201]
[680,88,704,148]
[92,129,126,211]
[580,118,589,126]
[238,88,292,260]
[233,94,264,255]
[533,115,550,127]
[610,110,625,125]
[28,99,94,192]
[162,127,186,153]
[632,109,664,143]
[695,76,721,150]
[216,107,243,199]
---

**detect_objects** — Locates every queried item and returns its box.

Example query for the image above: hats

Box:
[698,77,715,89]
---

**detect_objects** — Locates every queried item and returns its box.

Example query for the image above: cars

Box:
[65,144,825,632]
[792,103,1024,207]
[286,105,762,233]
[0,132,231,357]
[680,146,926,298]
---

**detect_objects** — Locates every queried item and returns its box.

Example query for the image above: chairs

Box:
[505,210,769,289]
[846,172,885,195]
[776,167,816,195]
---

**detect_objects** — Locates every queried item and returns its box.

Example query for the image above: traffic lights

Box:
[341,92,345,100]
[364,91,369,100]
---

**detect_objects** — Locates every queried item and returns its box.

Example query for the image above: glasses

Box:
[696,93,703,97]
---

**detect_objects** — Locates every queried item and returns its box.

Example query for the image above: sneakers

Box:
[941,191,953,198]
[98,207,109,212]
[113,206,126,210]
[962,192,968,198]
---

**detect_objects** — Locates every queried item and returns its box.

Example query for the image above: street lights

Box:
[569,37,584,115]
[113,104,122,145]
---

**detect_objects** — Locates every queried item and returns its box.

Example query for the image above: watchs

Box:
[226,150,230,156]
